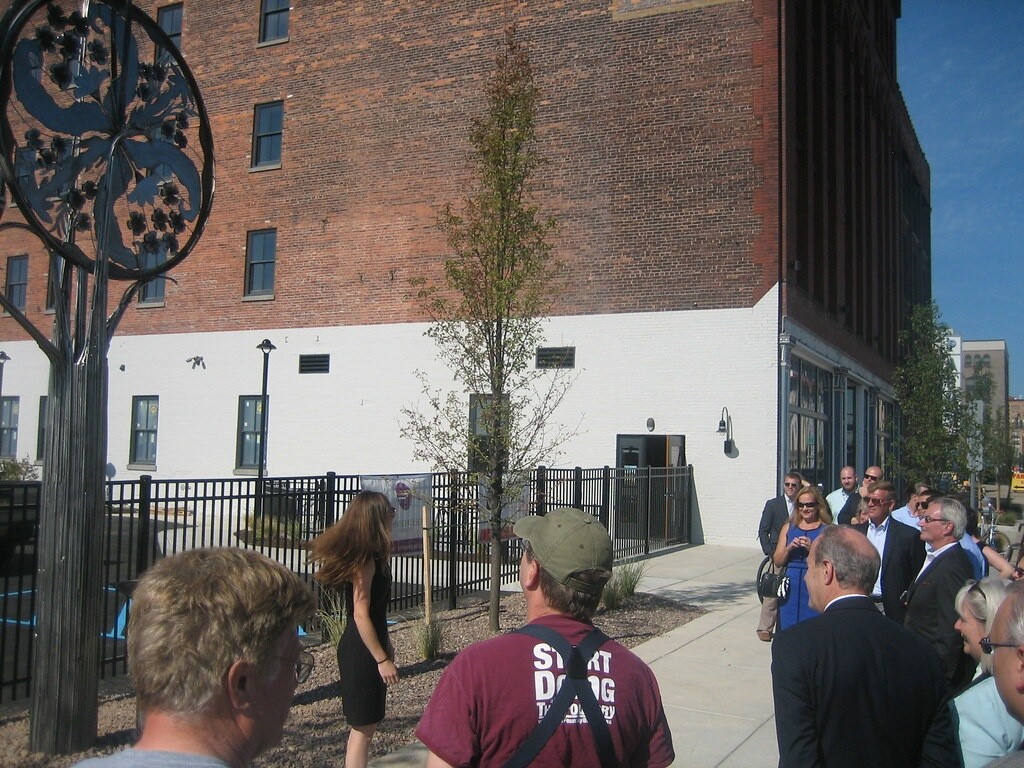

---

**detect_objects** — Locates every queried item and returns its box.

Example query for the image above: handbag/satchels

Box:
[760,559,791,600]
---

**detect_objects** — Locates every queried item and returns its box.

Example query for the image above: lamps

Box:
[186,356,206,369]
[716,406,733,453]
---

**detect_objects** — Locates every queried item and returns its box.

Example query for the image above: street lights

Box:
[0,351,13,443]
[253,338,277,523]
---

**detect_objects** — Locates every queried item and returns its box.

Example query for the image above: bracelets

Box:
[378,658,388,664]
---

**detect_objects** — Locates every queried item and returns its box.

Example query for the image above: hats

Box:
[513,507,614,596]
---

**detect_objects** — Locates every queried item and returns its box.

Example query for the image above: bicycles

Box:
[754,556,787,606]
[978,503,1014,564]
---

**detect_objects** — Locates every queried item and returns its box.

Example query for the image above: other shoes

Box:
[757,631,771,642]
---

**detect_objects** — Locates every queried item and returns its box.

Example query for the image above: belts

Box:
[871,597,883,603]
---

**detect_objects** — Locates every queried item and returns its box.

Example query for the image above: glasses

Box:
[964,579,986,601]
[262,651,314,683]
[784,482,797,488]
[863,496,892,504]
[798,502,819,508]
[1015,566,1024,578]
[914,501,929,510]
[864,473,881,481]
[978,637,1020,654]
[389,506,397,519]
[518,538,528,552]
[919,515,949,524]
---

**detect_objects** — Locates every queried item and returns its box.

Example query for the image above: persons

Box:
[837,466,885,526]
[414,508,675,768]
[774,486,833,630]
[964,507,1012,579]
[891,480,932,531]
[978,579,1024,768]
[71,550,315,767]
[303,491,401,768]
[901,490,988,697]
[757,471,817,642]
[1012,556,1024,580]
[853,481,928,623]
[771,524,965,768]
[825,466,862,525]
